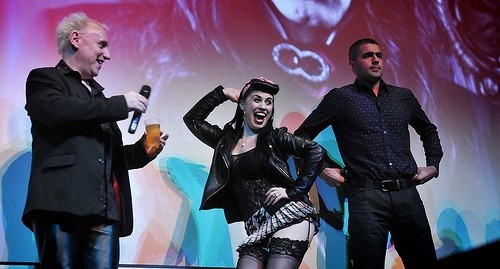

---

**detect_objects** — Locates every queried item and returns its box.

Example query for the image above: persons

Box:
[292,37,444,269]
[21,11,170,269]
[182,77,326,269]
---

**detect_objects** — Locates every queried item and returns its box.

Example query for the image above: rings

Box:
[273,192,277,197]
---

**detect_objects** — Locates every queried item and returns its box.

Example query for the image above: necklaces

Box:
[240,136,246,150]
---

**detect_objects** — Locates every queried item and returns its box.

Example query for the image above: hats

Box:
[240,77,280,100]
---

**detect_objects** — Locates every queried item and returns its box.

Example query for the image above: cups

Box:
[144,119,160,147]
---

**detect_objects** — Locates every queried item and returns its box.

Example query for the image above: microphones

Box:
[128,85,151,134]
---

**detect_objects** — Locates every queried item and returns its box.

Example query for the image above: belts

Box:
[349,174,415,192]
